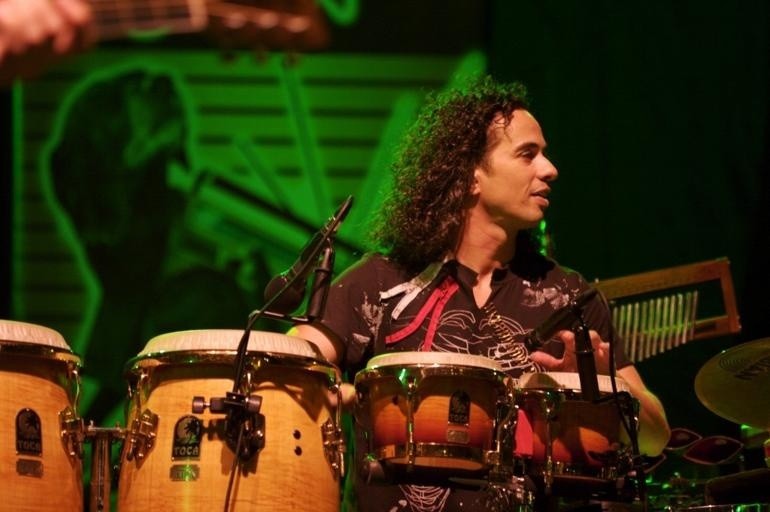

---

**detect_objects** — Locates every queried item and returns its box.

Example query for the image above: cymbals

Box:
[694,336,770,432]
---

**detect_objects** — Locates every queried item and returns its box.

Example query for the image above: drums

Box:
[114,329,347,512]
[508,370,633,478]
[355,351,511,476]
[0,319,84,512]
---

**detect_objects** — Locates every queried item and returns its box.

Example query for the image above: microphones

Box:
[265,195,354,313]
[525,288,595,354]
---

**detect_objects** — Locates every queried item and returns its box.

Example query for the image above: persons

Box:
[0,0,94,63]
[281,69,671,511]
